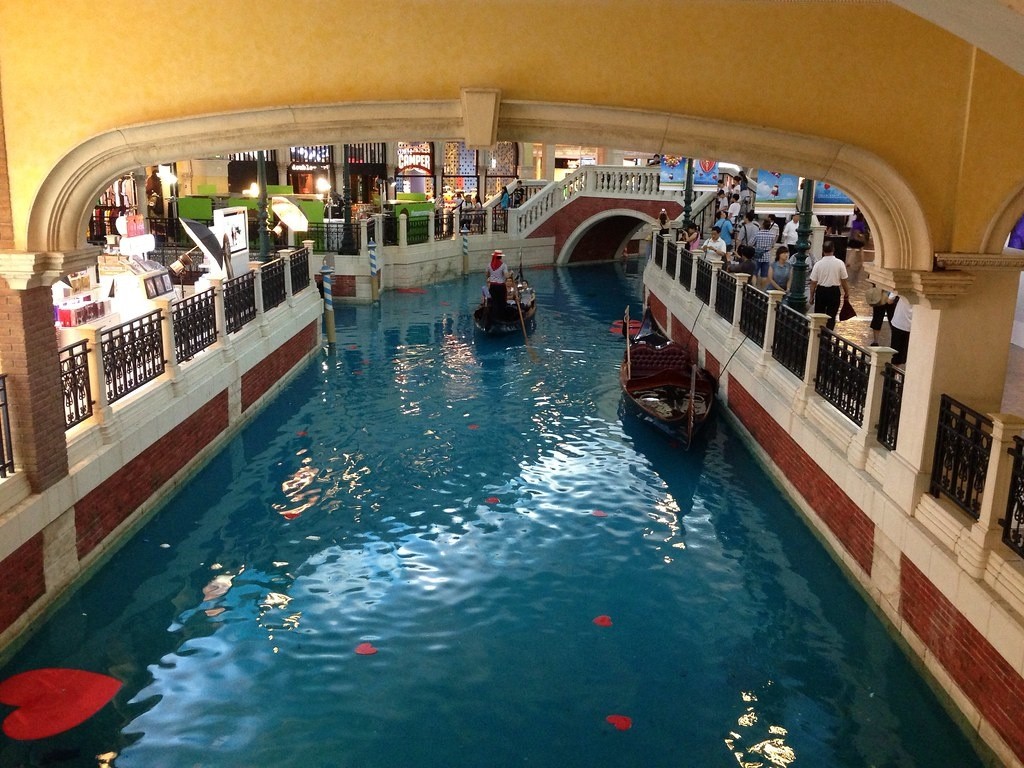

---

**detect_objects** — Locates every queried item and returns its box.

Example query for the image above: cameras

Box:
[679,230,684,233]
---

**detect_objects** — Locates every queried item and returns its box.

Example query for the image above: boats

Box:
[619,302,714,452]
[472,246,538,336]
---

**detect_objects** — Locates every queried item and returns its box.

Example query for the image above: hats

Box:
[491,250,505,256]
[717,190,724,195]
[741,246,755,259]
[776,246,789,255]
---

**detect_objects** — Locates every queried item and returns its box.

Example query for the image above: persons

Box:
[863,286,914,382]
[809,241,849,347]
[500,180,524,210]
[485,251,535,319]
[678,171,800,310]
[451,192,482,233]
[646,154,661,191]
[846,205,870,269]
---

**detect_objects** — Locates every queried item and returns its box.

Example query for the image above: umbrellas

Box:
[271,196,309,233]
[178,217,224,272]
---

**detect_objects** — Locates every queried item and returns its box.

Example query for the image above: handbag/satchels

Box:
[839,295,857,321]
[753,248,763,258]
[866,288,888,307]
[737,244,746,257]
[685,241,691,250]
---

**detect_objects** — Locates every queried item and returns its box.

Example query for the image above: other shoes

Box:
[880,371,899,381]
[870,342,879,346]
